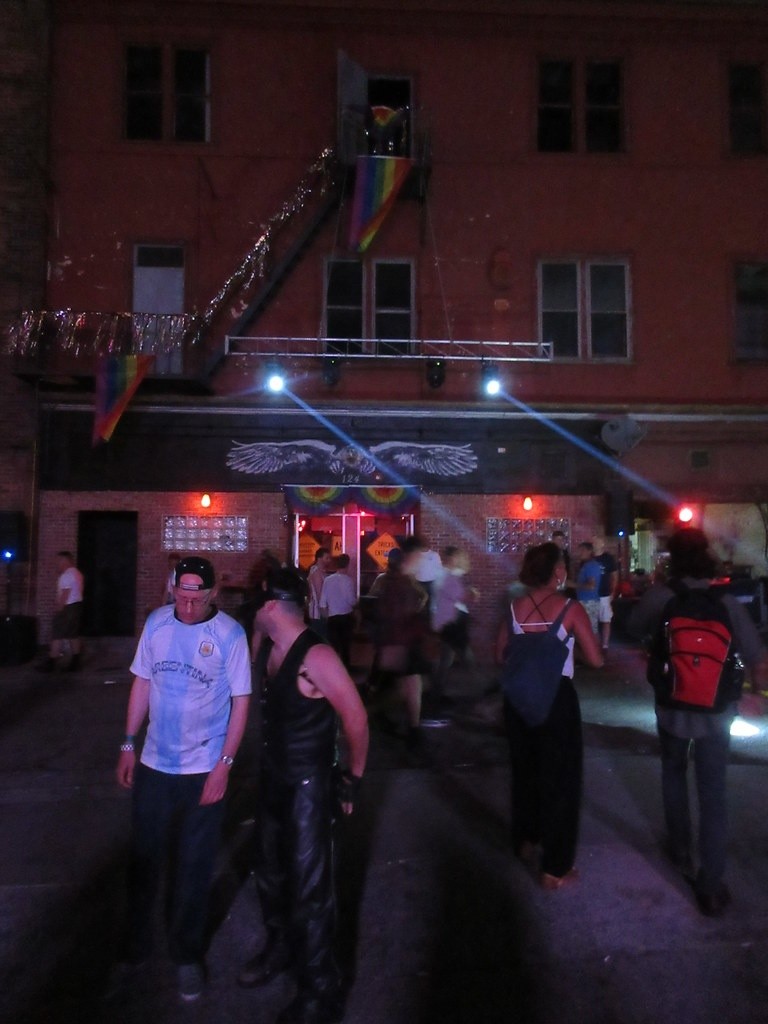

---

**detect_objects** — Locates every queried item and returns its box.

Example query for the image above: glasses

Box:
[171,587,212,606]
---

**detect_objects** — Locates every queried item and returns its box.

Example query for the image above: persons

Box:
[554,533,618,649]
[369,537,467,747]
[161,553,180,606]
[498,542,605,889]
[116,556,251,1000]
[628,531,768,916]
[238,574,369,1024]
[36,551,83,672]
[307,549,357,668]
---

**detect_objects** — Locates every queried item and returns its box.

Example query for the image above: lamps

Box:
[262,360,285,393]
[482,364,501,399]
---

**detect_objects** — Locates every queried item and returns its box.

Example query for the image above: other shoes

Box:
[515,836,534,863]
[275,991,340,1024]
[691,873,730,920]
[394,723,425,748]
[236,937,294,990]
[542,863,577,889]
[61,662,83,672]
[35,661,57,671]
[179,960,205,1001]
[96,960,146,999]
[661,836,692,868]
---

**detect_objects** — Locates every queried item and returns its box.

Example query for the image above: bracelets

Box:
[122,735,138,741]
[120,744,137,750]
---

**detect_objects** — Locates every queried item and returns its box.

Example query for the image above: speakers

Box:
[605,490,635,536]
[0,511,27,562]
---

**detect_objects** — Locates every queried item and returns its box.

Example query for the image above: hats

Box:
[172,557,216,590]
[256,567,309,602]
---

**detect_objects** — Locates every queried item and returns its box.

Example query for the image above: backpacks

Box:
[494,596,578,726]
[649,575,745,709]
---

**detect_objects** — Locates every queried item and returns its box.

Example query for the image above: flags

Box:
[93,354,156,443]
[349,157,413,252]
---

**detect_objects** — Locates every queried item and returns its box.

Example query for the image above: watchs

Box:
[220,755,235,765]
